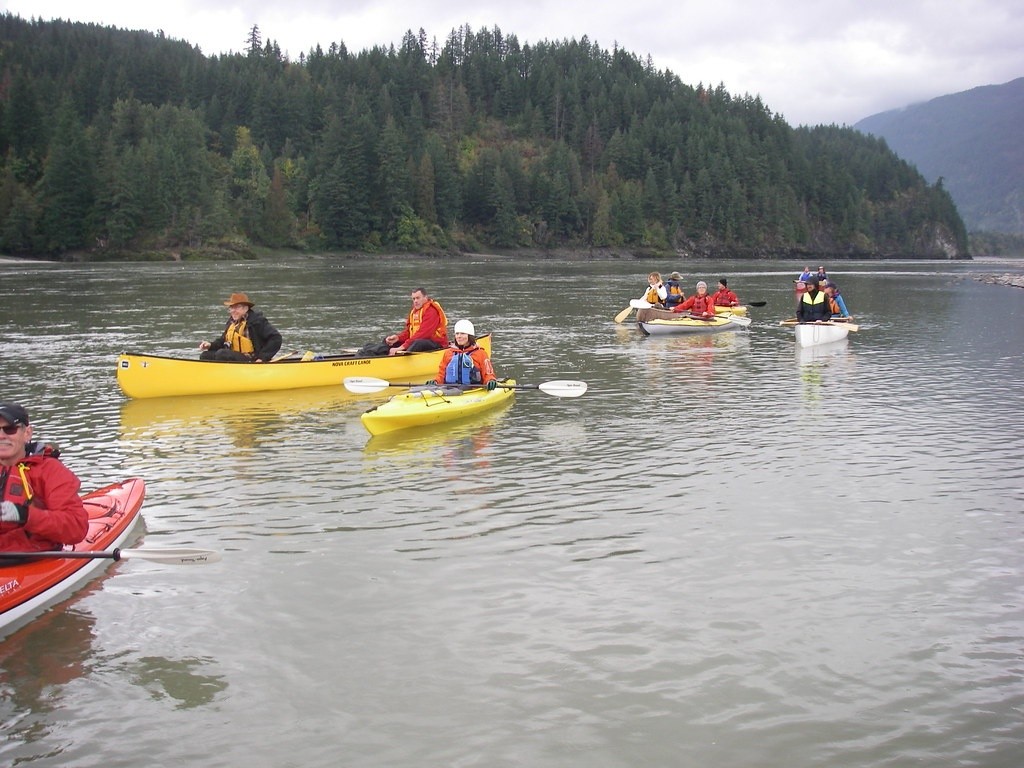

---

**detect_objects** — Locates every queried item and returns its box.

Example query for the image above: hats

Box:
[670,272,683,281]
[454,319,474,335]
[696,280,707,290]
[0,403,29,426]
[224,293,254,307]
[720,279,726,287]
[825,282,836,289]
[818,266,825,272]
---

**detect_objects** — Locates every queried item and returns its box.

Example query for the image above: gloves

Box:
[426,380,438,386]
[487,380,496,391]
[0,501,21,523]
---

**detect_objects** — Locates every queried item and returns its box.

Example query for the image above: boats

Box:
[361,377,517,436]
[117,332,492,399]
[0,477,145,642]
[638,305,746,335]
[794,316,849,347]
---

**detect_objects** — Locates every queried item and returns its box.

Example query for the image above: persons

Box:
[664,271,686,310]
[824,283,853,322]
[385,287,449,356]
[0,404,88,554]
[641,272,667,308]
[816,266,827,282]
[669,281,716,321]
[798,266,813,281]
[199,294,282,363]
[711,279,739,308]
[796,276,832,324]
[426,320,497,391]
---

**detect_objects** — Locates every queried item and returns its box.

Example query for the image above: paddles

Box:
[0,547,220,566]
[614,281,664,323]
[794,280,806,283]
[734,301,767,307]
[780,320,859,333]
[785,317,848,322]
[340,348,434,357]
[630,299,752,327]
[343,376,588,398]
[268,351,299,362]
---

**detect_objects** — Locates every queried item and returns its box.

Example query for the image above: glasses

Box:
[228,305,245,312]
[0,423,23,435]
